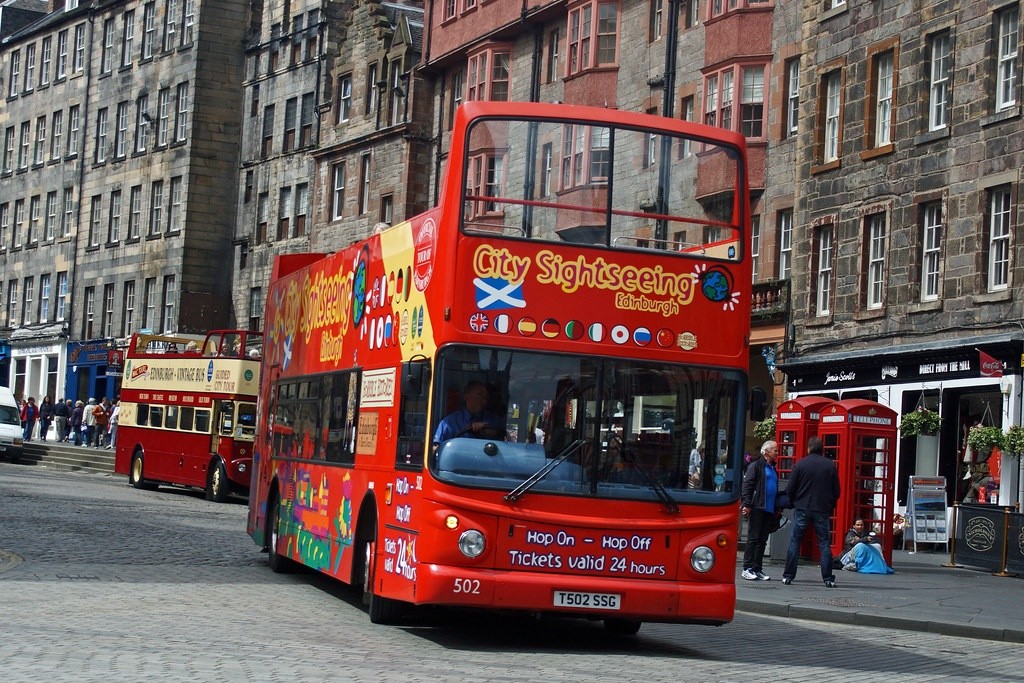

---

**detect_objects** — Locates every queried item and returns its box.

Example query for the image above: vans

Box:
[0,386,27,463]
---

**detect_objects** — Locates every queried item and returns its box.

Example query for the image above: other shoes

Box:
[826,580,837,587]
[782,577,792,584]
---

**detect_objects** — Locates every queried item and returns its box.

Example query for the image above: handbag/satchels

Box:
[80,421,89,435]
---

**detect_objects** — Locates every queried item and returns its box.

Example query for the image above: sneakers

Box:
[742,568,771,581]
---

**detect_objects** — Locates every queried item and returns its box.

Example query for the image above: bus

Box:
[242,100,768,636]
[112,329,264,502]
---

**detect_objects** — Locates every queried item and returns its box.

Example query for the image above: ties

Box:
[471,415,480,439]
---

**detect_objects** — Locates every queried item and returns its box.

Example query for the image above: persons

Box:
[782,437,841,588]
[688,443,754,492]
[741,441,778,580]
[527,424,543,444]
[505,430,517,442]
[840,518,894,574]
[169,341,260,358]
[13,397,121,449]
[433,382,512,467]
[873,514,905,534]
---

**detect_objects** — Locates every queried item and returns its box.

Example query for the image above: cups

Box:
[868,531,876,541]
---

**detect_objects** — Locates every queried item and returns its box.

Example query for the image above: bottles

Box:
[970,476,997,505]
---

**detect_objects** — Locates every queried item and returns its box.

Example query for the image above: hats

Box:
[89,397,96,402]
